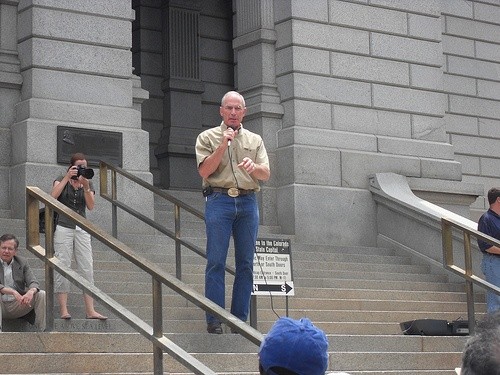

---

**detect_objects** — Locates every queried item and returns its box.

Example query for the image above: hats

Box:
[258,317,330,375]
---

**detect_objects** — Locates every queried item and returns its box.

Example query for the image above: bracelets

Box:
[84,188,90,192]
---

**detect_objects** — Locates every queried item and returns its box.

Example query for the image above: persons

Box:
[460,312,500,375]
[195,91,270,333]
[258,316,329,375]
[51,154,108,320]
[0,234,46,331]
[477,187,500,311]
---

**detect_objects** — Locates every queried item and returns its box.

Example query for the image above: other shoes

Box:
[61,312,71,319]
[86,313,108,320]
[230,328,239,334]
[208,324,223,334]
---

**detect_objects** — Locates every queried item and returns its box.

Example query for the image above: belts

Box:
[210,187,254,197]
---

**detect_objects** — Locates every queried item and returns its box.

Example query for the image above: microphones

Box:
[227,126,233,147]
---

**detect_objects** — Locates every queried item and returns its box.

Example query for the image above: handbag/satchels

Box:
[38,206,58,234]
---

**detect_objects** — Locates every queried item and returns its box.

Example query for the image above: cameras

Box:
[71,165,94,180]
